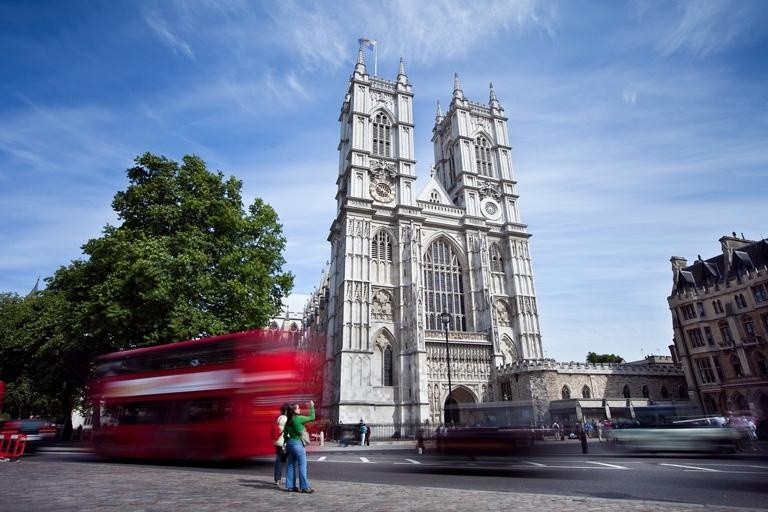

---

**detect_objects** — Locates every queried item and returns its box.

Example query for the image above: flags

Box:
[358,36,376,52]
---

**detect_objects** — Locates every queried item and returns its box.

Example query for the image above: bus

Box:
[88,328,327,468]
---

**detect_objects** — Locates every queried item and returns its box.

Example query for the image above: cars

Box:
[603,411,758,462]
[0,418,59,458]
[416,420,552,460]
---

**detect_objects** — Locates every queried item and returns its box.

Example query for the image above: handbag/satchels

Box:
[299,431,310,447]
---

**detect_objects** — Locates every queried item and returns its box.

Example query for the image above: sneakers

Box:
[274,480,280,488]
[288,487,299,491]
[301,488,314,493]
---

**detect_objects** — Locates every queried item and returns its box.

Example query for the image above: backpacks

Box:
[270,414,283,441]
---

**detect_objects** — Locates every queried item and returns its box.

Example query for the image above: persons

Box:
[415,428,425,454]
[729,413,760,451]
[365,426,371,446]
[433,420,550,439]
[282,400,317,492]
[272,402,291,486]
[360,424,367,446]
[551,419,613,454]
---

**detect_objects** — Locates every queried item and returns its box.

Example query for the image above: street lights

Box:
[438,309,455,426]
